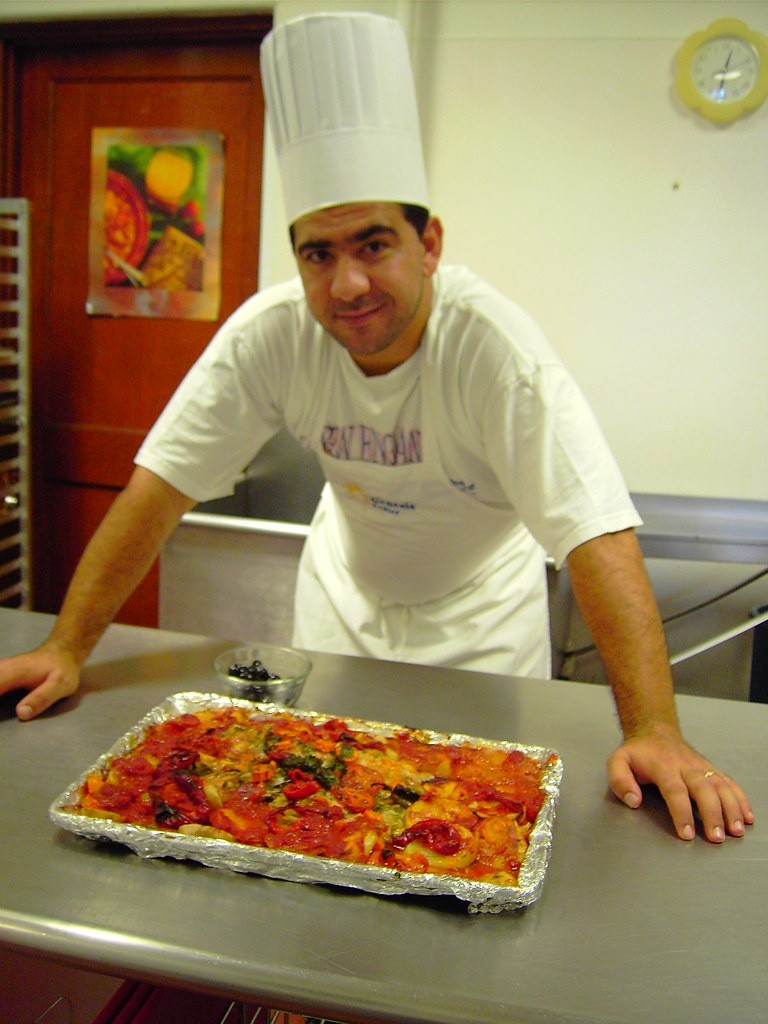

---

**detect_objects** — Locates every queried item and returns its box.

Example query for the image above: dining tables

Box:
[0,605,768,1024]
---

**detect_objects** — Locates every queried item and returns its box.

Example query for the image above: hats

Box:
[259,12,431,229]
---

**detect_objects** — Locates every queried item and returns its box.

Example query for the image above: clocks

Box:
[675,17,768,124]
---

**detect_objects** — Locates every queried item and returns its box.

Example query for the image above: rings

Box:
[704,771,714,777]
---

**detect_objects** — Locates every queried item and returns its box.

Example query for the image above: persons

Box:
[0,198,755,842]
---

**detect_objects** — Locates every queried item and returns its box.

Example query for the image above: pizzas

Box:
[59,706,545,891]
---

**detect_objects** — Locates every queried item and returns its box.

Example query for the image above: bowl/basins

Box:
[216,644,312,707]
